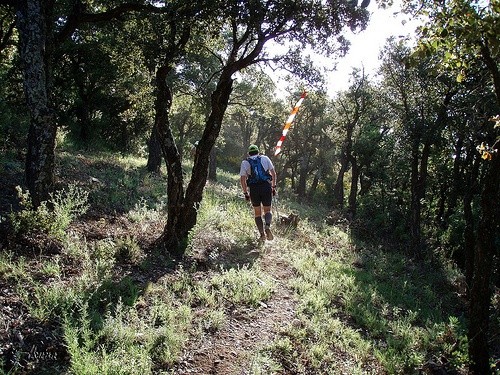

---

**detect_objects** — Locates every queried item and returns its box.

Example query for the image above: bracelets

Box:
[243,191,249,195]
[272,184,277,188]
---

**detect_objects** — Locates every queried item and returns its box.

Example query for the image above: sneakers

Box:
[259,232,266,240]
[265,226,273,240]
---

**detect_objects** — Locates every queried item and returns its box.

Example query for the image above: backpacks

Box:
[247,155,272,186]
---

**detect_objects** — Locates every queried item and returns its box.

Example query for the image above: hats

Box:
[248,145,258,152]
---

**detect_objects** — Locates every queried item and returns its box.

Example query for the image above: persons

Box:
[239,143,278,240]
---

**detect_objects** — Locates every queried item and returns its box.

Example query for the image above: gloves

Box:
[273,187,277,196]
[243,191,250,202]
